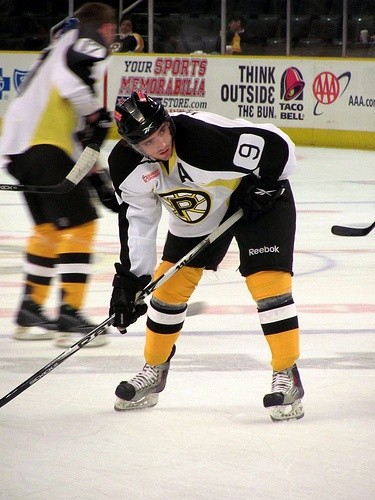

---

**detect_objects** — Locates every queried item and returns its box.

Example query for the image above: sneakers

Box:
[57,304,108,348]
[114,345,176,410]
[263,363,305,423]
[12,300,57,340]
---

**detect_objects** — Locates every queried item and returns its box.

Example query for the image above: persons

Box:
[112,15,144,53]
[0,3,117,347]
[215,12,264,56]
[108,91,305,422]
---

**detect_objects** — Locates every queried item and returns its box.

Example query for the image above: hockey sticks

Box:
[0,143,101,195]
[331,221,375,237]
[0,206,245,406]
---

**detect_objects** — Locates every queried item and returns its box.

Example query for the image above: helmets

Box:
[114,91,169,145]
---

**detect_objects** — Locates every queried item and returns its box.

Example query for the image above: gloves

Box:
[109,262,152,334]
[86,107,113,129]
[239,184,278,223]
[88,168,120,214]
